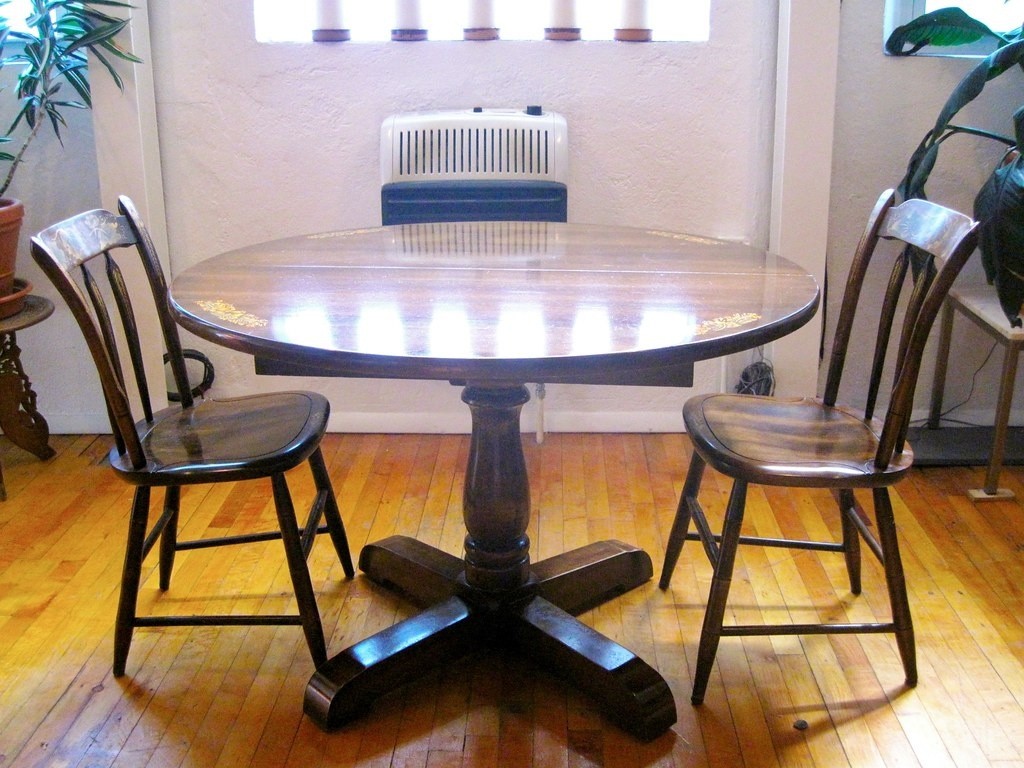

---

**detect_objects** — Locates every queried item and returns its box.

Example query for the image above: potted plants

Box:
[0,0,145,319]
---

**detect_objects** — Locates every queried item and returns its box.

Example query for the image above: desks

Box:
[926,272,1024,503]
[0,293,56,502]
[165,221,821,743]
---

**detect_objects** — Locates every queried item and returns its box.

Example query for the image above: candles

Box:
[393,0,423,29]
[467,0,497,28]
[311,0,344,29]
[549,0,579,28]
[616,0,651,29]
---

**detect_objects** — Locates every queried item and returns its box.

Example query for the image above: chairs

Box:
[657,187,986,708]
[30,190,355,678]
